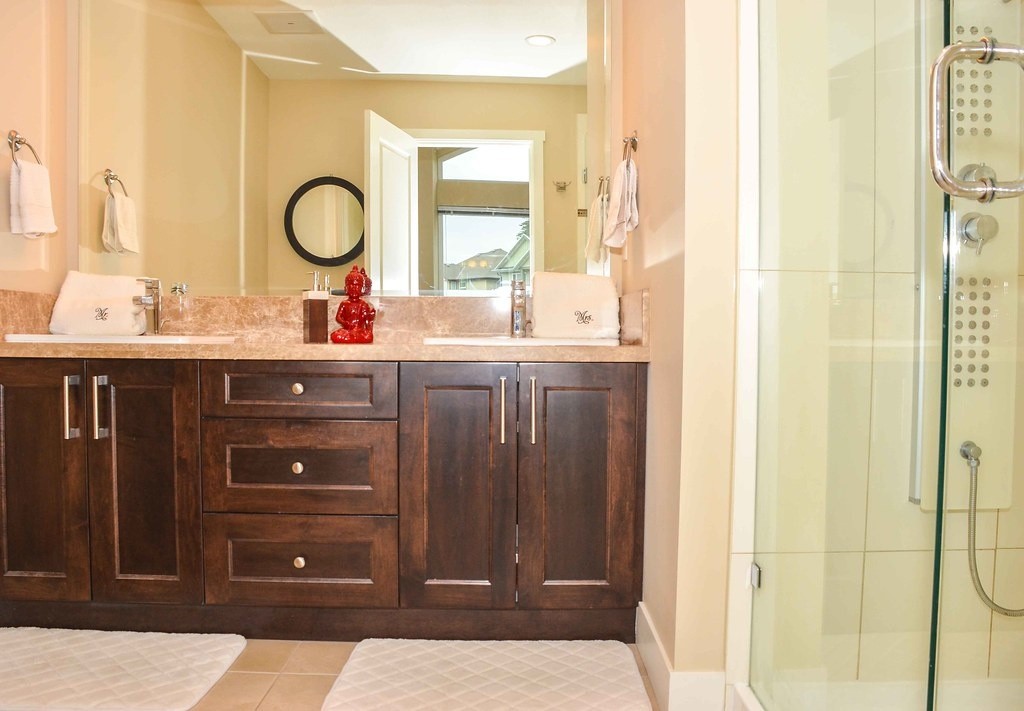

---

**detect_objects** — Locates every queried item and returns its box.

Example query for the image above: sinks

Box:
[3,333,235,344]
[422,336,620,348]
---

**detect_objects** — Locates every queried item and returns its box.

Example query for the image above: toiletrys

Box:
[323,273,333,297]
[302,270,329,346]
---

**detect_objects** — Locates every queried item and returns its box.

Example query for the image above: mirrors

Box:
[284,176,364,267]
[68,0,611,298]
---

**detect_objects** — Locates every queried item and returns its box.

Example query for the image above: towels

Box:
[531,271,622,339]
[9,157,58,241]
[602,158,639,249]
[48,269,148,337]
[100,190,140,256]
[584,192,609,264]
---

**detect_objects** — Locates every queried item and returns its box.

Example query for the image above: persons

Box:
[331,267,375,343]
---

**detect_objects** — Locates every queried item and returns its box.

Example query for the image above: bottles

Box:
[167,283,190,335]
[511,271,527,338]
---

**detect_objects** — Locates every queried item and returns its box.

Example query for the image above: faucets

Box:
[510,279,532,339]
[170,281,190,297]
[132,278,160,337]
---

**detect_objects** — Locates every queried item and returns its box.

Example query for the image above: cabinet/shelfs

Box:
[0,356,205,605]
[200,358,399,610]
[398,361,637,612]
